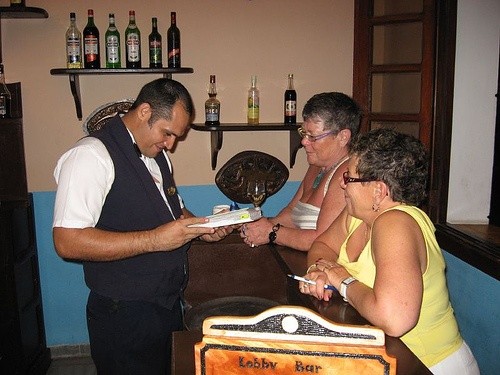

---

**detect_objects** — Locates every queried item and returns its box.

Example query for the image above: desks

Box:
[171,232,434,375]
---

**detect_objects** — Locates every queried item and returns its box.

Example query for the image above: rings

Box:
[304,285,307,287]
[299,286,303,289]
[331,265,333,267]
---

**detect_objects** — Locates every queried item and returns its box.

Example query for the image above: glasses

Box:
[343,171,383,185]
[298,127,334,142]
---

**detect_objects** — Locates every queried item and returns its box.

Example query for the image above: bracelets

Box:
[269,223,280,245]
[306,264,316,274]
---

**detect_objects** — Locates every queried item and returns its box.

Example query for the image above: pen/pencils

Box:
[287,274,336,291]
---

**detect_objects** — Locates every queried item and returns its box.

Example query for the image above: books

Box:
[188,207,262,227]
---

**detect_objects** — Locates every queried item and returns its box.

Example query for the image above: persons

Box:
[299,128,480,375]
[238,92,362,251]
[51,78,243,375]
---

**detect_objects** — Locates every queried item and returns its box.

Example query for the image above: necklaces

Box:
[312,173,323,188]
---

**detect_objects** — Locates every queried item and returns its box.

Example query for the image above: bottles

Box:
[81,9,100,69]
[205,75,220,126]
[148,17,162,68]
[65,12,83,69]
[124,10,141,69]
[283,73,296,124]
[247,76,260,124]
[105,13,121,68]
[167,11,181,68]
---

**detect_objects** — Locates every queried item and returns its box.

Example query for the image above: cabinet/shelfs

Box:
[0,5,304,170]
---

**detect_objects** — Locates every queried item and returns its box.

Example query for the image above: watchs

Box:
[341,277,355,299]
